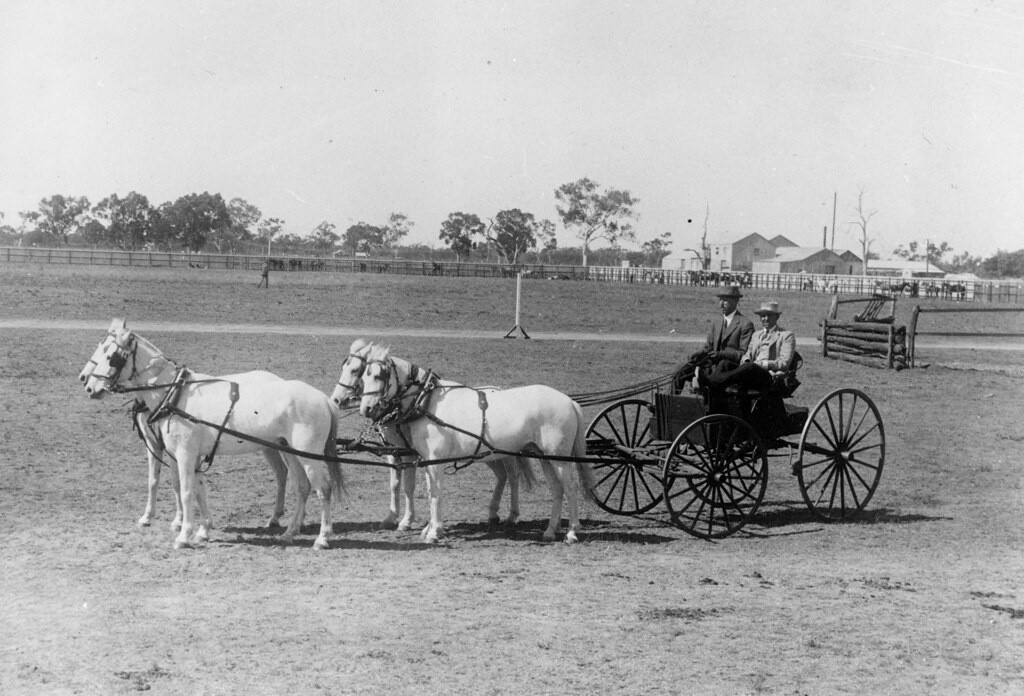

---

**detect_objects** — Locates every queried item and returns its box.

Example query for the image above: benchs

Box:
[725,352,803,398]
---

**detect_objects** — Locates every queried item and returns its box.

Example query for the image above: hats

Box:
[754,302,782,315]
[716,286,743,298]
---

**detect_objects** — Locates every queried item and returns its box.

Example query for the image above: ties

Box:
[724,320,727,329]
[761,329,769,341]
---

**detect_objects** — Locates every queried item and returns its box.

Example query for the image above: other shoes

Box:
[692,366,707,391]
[706,411,710,415]
[704,416,726,423]
[647,404,656,413]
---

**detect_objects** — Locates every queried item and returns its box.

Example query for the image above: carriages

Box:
[79,316,886,550]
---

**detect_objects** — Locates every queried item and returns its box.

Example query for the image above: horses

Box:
[332,339,600,545]
[79,316,344,553]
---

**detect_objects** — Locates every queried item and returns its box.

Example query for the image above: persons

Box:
[817,275,838,293]
[701,285,754,380]
[691,301,796,427]
